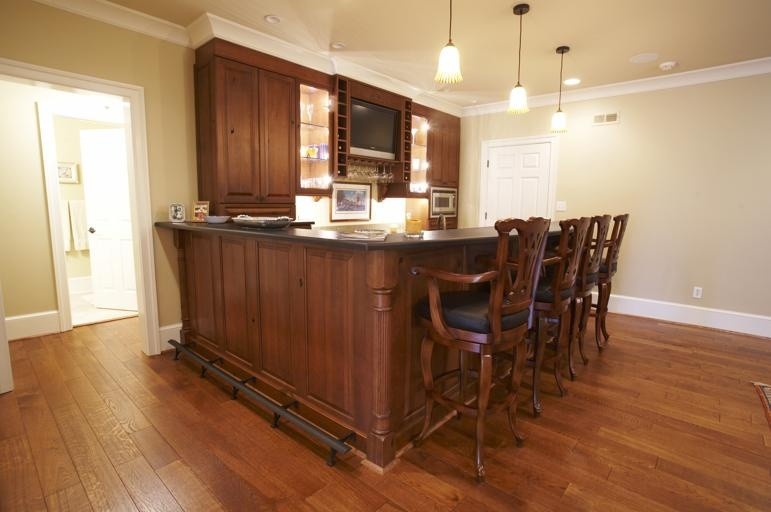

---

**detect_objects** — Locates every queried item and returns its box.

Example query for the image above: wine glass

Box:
[347,157,394,185]
[300,102,323,125]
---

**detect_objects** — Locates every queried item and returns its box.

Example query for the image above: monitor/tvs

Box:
[350,98,398,160]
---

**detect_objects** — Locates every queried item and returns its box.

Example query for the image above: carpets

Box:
[751,381,771,432]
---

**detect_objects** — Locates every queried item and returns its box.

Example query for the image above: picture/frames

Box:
[57,163,79,184]
[330,181,372,222]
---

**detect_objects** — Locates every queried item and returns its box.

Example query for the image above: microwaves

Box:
[428,186,457,218]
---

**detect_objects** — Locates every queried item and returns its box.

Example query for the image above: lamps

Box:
[507,3,530,116]
[551,46,570,131]
[434,1,463,83]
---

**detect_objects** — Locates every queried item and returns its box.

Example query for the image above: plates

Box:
[231,216,294,230]
[203,215,230,224]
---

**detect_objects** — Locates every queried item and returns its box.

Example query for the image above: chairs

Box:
[590,213,629,353]
[475,215,590,416]
[409,214,551,485]
[563,214,611,382]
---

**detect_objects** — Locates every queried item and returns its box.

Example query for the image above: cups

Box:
[404,218,421,233]
[300,143,329,160]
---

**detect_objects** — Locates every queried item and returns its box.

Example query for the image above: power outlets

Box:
[692,286,703,299]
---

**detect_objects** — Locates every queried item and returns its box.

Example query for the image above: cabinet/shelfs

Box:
[380,101,459,230]
[176,230,251,388]
[334,73,411,186]
[296,63,335,198]
[192,37,296,221]
[253,239,356,434]
